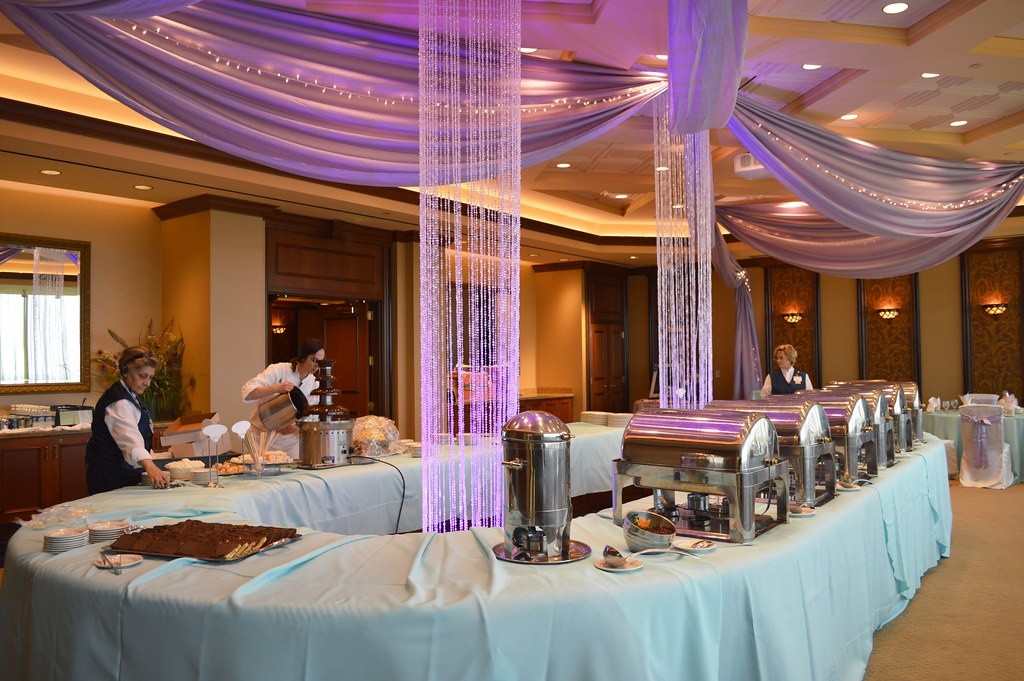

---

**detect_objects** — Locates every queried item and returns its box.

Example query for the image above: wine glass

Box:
[250,455,266,480]
[942,399,958,414]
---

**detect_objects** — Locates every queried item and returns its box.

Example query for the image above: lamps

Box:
[982,304,1008,321]
[271,325,287,334]
[877,308,902,324]
[782,313,803,325]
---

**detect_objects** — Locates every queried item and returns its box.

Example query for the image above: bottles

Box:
[9,404,51,416]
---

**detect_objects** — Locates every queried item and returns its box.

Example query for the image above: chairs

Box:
[959,404,1014,490]
[958,393,1000,405]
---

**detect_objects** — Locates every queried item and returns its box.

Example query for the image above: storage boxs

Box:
[160,412,231,458]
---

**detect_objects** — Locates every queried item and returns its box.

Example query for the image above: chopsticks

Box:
[244,429,280,473]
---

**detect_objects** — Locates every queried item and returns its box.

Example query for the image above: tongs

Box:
[101,551,121,576]
[154,483,185,489]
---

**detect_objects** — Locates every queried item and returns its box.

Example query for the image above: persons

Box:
[85,346,170,496]
[241,338,325,460]
[760,344,813,400]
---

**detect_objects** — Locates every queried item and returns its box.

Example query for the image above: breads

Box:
[110,519,296,559]
[214,450,292,475]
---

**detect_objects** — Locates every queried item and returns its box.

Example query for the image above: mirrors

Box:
[0,233,92,395]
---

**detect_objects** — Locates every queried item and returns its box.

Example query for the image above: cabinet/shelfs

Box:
[446,398,573,437]
[0,420,175,545]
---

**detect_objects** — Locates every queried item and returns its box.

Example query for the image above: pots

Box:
[51,405,94,427]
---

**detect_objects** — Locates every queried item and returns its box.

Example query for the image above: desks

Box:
[922,409,1024,484]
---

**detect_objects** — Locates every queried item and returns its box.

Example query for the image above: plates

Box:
[398,439,414,454]
[581,411,634,427]
[786,482,861,515]
[435,433,453,445]
[456,433,479,446]
[141,471,171,486]
[594,538,717,572]
[405,442,422,458]
[42,528,89,554]
[192,469,218,485]
[95,554,143,569]
[226,461,296,465]
[88,521,131,544]
[1015,407,1023,414]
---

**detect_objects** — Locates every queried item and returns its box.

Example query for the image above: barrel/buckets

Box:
[258,386,309,431]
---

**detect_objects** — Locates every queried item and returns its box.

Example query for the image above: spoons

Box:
[691,540,753,549]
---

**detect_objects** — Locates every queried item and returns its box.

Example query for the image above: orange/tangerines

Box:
[636,517,651,528]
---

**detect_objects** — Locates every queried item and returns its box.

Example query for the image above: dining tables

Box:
[0,423,952,681]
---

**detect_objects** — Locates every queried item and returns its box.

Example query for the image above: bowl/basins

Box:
[165,458,205,480]
[624,510,676,555]
